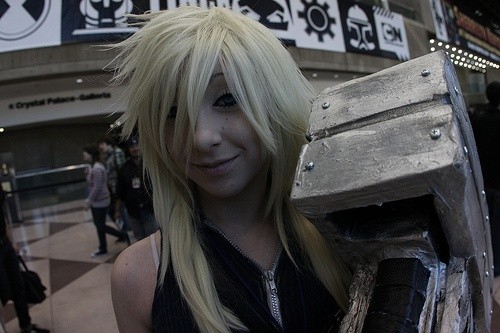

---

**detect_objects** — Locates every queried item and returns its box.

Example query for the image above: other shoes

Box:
[113,238,126,244]
[93,248,108,256]
[19,323,50,333]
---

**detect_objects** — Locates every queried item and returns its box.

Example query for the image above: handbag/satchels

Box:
[14,257,49,305]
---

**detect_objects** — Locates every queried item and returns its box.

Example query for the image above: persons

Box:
[104,5,500,333]
[0,135,155,333]
[466,82,500,276]
